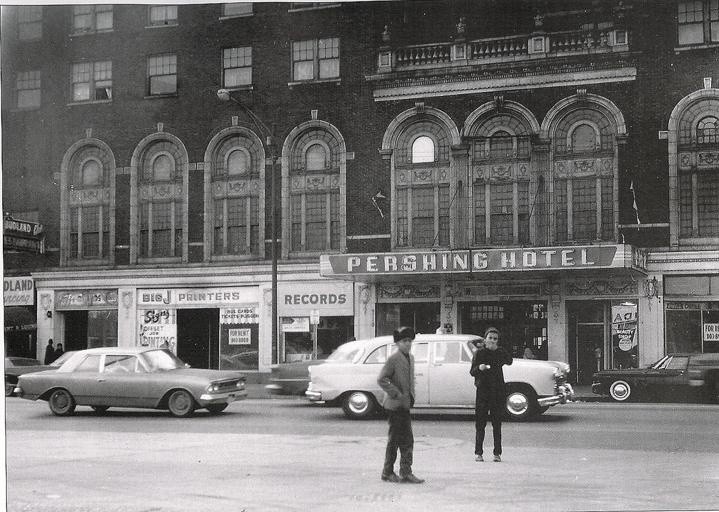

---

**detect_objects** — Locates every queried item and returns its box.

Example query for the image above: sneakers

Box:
[474,453,485,462]
[492,454,502,462]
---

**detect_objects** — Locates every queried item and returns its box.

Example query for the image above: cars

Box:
[220,349,259,371]
[592,353,719,401]
[12,346,247,417]
[573,335,602,376]
[307,332,575,418]
[268,341,369,407]
[5,350,80,396]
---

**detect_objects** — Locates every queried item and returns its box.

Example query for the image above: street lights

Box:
[213,87,280,371]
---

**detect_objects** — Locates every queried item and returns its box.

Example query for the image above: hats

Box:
[393,326,415,342]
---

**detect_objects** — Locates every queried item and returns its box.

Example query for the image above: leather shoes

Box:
[398,474,425,484]
[379,471,402,483]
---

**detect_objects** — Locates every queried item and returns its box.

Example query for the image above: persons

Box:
[469,327,513,462]
[44,339,56,364]
[55,343,64,359]
[375,327,424,484]
